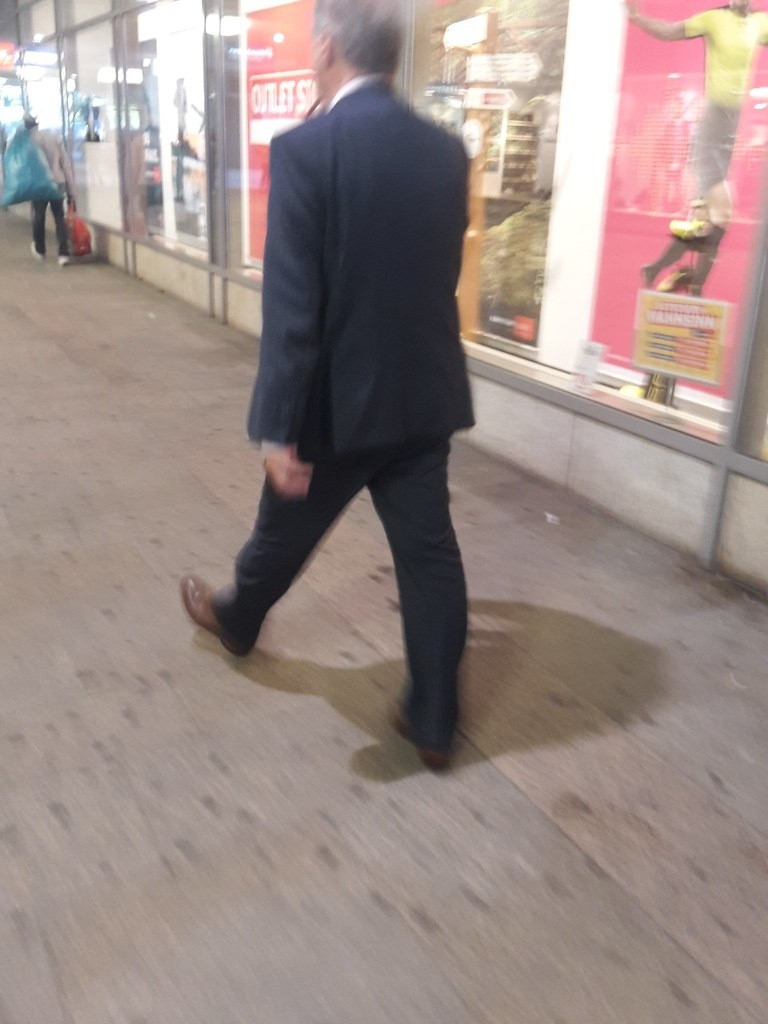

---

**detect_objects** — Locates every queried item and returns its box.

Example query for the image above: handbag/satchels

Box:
[64,202,90,257]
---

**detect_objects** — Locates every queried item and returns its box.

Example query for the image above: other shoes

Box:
[59,255,70,266]
[31,241,44,259]
[388,700,448,764]
[182,574,251,657]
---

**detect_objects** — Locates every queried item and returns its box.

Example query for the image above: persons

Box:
[21,111,78,265]
[178,0,475,773]
[628,0,766,296]
[82,120,101,143]
